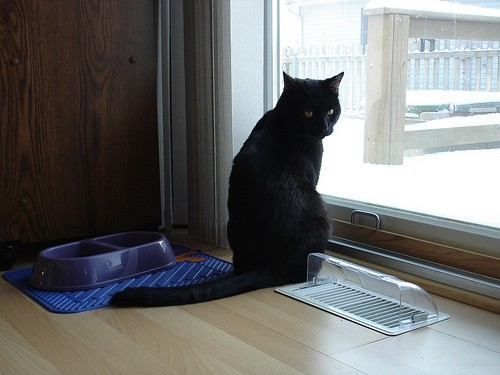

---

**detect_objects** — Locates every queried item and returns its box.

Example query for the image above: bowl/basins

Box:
[29,231,177,290]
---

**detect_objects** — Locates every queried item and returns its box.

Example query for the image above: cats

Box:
[109,70,345,308]
